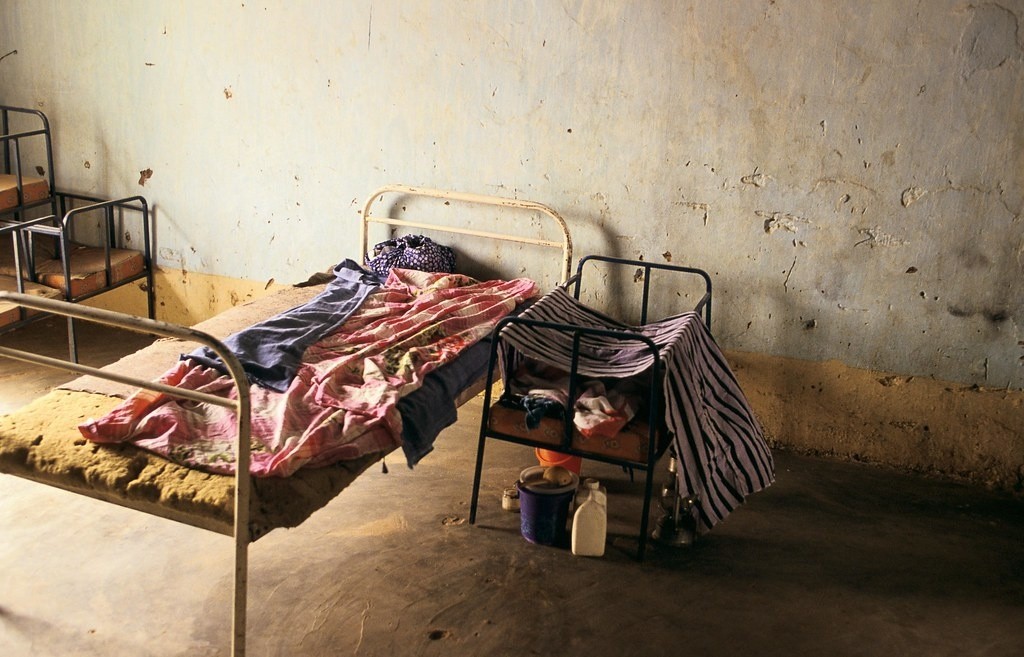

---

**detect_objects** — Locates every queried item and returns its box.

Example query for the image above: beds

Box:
[469,255,712,561]
[0,186,572,656]
[0,102,156,376]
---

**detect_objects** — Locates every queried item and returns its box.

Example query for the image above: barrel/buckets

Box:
[570,478,608,557]
[534,447,581,476]
[515,465,579,547]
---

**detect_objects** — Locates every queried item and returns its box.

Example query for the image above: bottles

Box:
[501,488,520,512]
